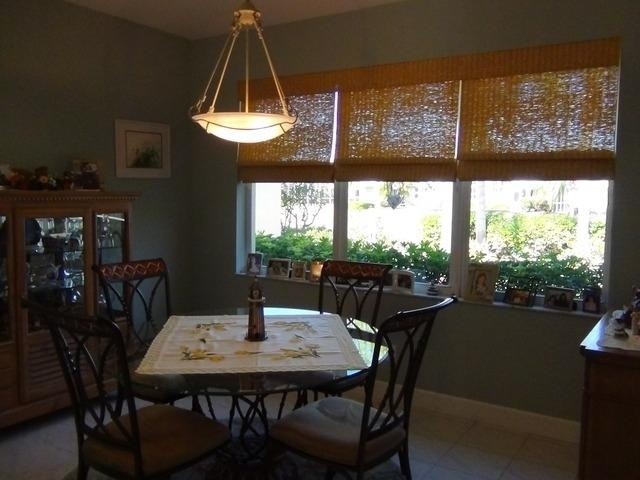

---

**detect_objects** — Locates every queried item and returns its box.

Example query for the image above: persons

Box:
[547,293,569,307]
[585,295,596,311]
[248,255,260,273]
[268,261,287,276]
[477,274,492,301]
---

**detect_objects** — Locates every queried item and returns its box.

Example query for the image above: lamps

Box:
[310,252,326,282]
[191,0,298,144]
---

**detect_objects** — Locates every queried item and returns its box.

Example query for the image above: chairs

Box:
[91,257,189,417]
[292,261,395,405]
[42,310,232,480]
[262,296,453,479]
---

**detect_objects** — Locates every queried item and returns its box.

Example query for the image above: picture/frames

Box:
[246,253,263,275]
[542,285,576,312]
[462,262,499,304]
[582,285,601,315]
[114,118,171,179]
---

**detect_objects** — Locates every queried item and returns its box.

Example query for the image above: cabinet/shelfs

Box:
[0,184,142,432]
[577,306,640,480]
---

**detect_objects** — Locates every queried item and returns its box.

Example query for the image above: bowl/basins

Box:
[40,236,65,249]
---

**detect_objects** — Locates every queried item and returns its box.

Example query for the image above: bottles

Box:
[247,275,262,299]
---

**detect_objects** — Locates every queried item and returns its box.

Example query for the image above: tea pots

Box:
[35,263,62,282]
